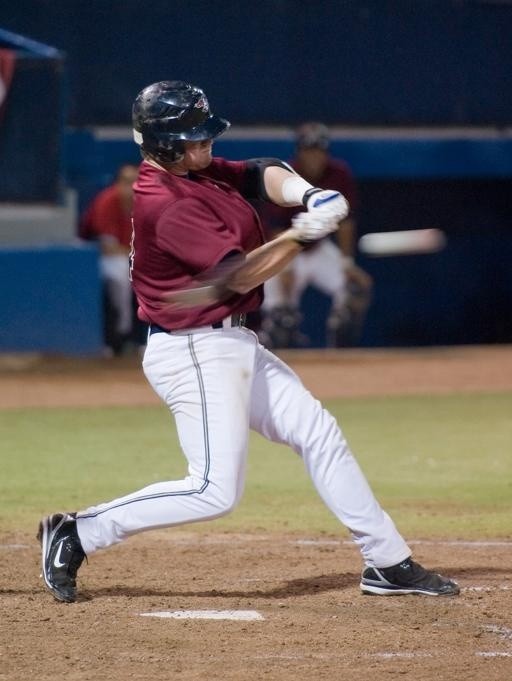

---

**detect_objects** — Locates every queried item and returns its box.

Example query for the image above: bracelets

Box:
[277,175,314,205]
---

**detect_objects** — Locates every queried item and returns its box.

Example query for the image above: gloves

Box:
[290,208,340,244]
[302,186,350,223]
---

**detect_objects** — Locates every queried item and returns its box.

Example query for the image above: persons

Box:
[252,120,374,347]
[35,82,461,604]
[80,163,141,358]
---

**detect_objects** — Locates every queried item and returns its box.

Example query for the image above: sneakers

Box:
[360,556,461,598]
[36,511,86,602]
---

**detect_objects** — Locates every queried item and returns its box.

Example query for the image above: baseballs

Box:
[415,229,442,252]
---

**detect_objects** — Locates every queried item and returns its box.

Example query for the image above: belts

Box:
[150,309,265,335]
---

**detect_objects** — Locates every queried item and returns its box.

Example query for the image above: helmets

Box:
[131,79,232,164]
[296,121,333,153]
